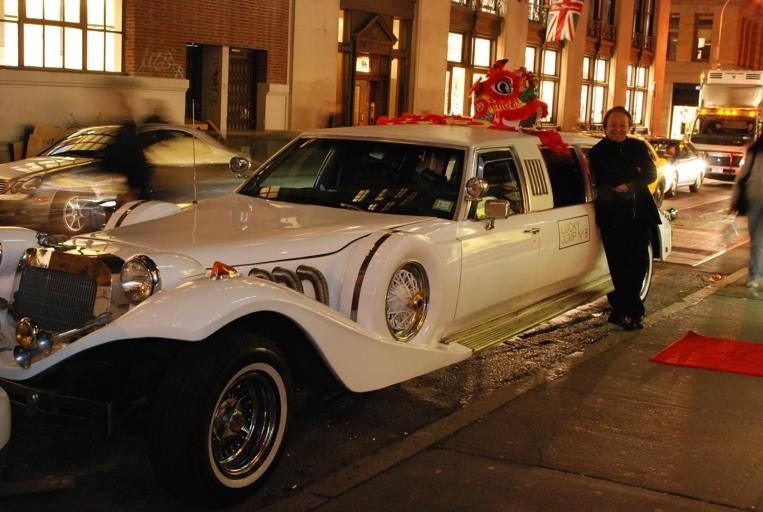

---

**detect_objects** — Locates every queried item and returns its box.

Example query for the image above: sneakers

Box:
[607,309,644,330]
[746,274,760,288]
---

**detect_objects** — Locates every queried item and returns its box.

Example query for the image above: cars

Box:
[0,120,678,492]
[589,129,706,207]
[0,123,264,233]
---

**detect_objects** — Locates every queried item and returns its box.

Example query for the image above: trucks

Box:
[686,70,762,184]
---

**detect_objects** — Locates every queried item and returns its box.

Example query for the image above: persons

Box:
[706,123,724,134]
[737,123,754,135]
[725,129,763,287]
[586,106,663,331]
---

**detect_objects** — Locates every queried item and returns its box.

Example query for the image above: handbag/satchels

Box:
[729,177,749,217]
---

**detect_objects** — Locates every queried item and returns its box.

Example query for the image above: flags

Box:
[544,0,584,43]
